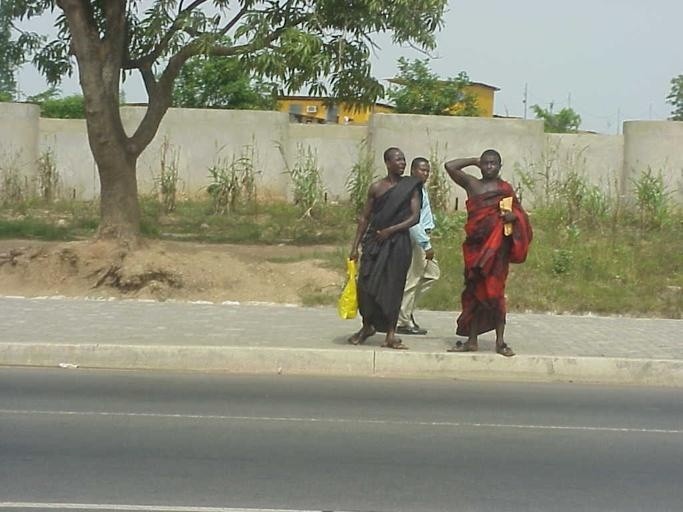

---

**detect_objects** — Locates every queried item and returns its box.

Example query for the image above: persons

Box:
[443,147,534,357]
[345,146,425,351]
[394,157,442,337]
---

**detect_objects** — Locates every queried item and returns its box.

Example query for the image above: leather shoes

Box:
[398,325,427,335]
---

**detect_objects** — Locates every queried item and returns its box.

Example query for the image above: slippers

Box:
[381,337,408,350]
[447,341,477,351]
[348,325,379,344]
[498,343,515,358]
